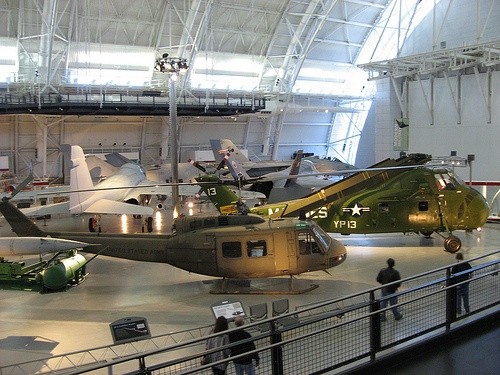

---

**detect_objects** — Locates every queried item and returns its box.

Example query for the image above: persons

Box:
[229,316,260,375]
[452,251,475,314]
[88,214,103,232]
[376,257,401,321]
[202,316,231,375]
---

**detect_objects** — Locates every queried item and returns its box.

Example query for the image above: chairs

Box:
[249,303,274,333]
[273,299,300,327]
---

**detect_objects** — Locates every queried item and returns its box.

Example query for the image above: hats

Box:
[456,253,463,260]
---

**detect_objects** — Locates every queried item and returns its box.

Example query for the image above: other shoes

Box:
[381,319,386,322]
[395,315,403,320]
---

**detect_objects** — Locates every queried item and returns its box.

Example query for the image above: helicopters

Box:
[0,139,492,301]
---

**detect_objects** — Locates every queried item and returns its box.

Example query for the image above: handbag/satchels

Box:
[201,348,210,365]
